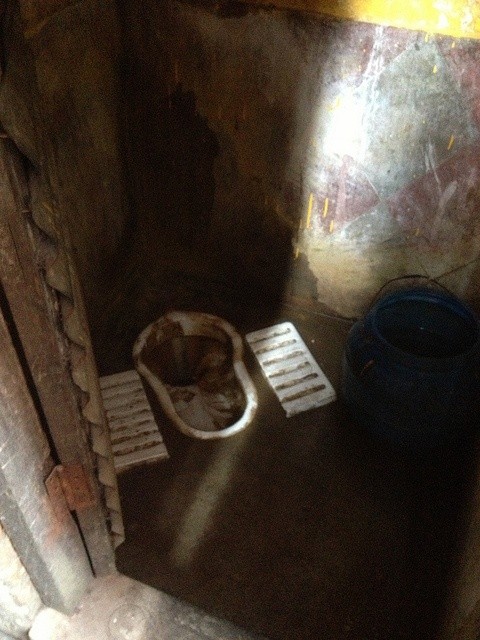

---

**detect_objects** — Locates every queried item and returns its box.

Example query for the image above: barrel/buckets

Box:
[340,288,480,462]
